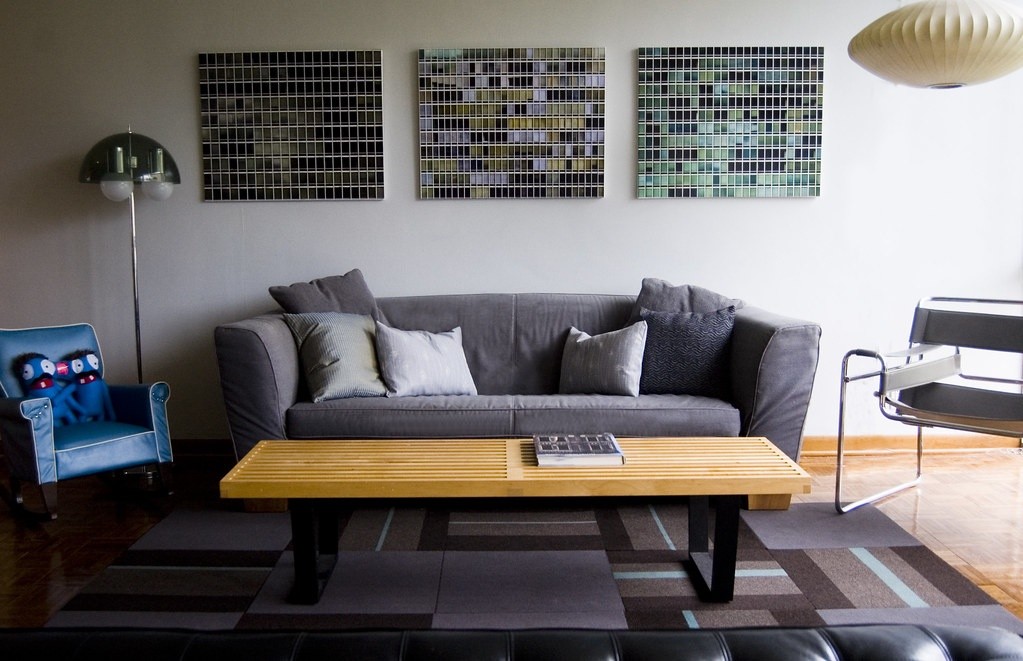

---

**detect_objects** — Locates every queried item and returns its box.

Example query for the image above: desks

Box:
[220,435,813,605]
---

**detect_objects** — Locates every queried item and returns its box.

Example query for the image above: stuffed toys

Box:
[19,352,116,430]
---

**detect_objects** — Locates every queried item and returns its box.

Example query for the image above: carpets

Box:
[42,500,1023,639]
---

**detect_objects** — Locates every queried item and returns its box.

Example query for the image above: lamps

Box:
[77,123,181,479]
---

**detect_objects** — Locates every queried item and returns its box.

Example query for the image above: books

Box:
[533,432,626,467]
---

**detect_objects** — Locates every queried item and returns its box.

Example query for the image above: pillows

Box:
[556,276,742,399]
[269,269,480,406]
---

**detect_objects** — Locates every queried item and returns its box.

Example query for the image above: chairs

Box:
[0,323,174,519]
[834,296,1023,515]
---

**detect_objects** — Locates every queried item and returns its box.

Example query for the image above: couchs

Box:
[214,268,822,512]
